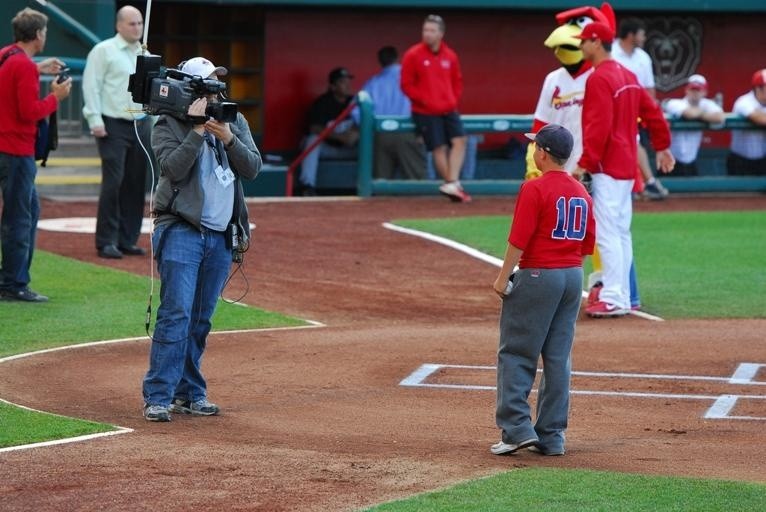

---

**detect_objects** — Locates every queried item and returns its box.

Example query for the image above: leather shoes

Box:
[119,244,145,254]
[99,244,122,258]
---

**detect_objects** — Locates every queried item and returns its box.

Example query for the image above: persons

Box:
[349,44,431,181]
[608,17,669,203]
[726,67,766,176]
[489,122,597,456]
[140,56,264,421]
[299,67,360,196]
[0,6,71,301]
[573,22,676,317]
[400,14,473,204]
[658,72,725,177]
[79,5,153,259]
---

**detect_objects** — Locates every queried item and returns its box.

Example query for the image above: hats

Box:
[330,69,354,84]
[524,124,573,158]
[572,23,613,44]
[544,3,616,65]
[687,74,708,92]
[752,69,766,87]
[182,57,228,79]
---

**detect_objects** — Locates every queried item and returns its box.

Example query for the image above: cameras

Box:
[56,67,72,83]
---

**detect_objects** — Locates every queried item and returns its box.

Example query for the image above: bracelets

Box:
[225,134,236,148]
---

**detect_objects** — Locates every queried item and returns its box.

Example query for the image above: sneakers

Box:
[528,445,565,457]
[0,289,48,301]
[439,181,471,203]
[142,403,171,422]
[491,438,539,455]
[641,180,668,200]
[584,302,643,318]
[168,398,220,416]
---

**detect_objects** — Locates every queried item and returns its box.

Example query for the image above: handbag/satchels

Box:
[36,117,49,162]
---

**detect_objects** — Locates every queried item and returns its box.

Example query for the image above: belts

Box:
[120,117,149,126]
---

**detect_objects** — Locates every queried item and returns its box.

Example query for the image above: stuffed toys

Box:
[526,4,616,305]
